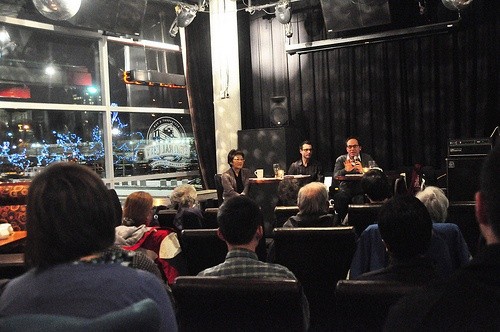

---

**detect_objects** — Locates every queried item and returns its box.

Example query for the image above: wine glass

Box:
[273,164,280,178]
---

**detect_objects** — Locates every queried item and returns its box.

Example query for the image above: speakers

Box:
[319,0,392,33]
[445,158,488,203]
[269,95,289,126]
[236,126,304,177]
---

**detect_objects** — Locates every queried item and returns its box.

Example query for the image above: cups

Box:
[362,168,369,174]
[368,160,375,169]
[254,169,263,179]
[277,170,284,179]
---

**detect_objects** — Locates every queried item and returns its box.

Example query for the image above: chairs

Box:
[157,174,500,332]
[0,182,31,267]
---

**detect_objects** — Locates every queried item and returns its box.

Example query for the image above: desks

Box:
[0,231,28,250]
[335,175,390,205]
[249,175,312,186]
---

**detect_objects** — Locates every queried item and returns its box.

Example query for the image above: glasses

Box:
[302,148,312,152]
[347,145,359,149]
[233,157,246,162]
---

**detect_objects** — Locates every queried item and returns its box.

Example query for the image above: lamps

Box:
[169,4,199,38]
[275,0,293,38]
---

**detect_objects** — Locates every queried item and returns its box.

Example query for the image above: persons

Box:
[334,137,379,203]
[288,141,323,186]
[222,149,251,199]
[196,197,310,330]
[170,184,203,228]
[0,162,191,332]
[278,135,500,332]
